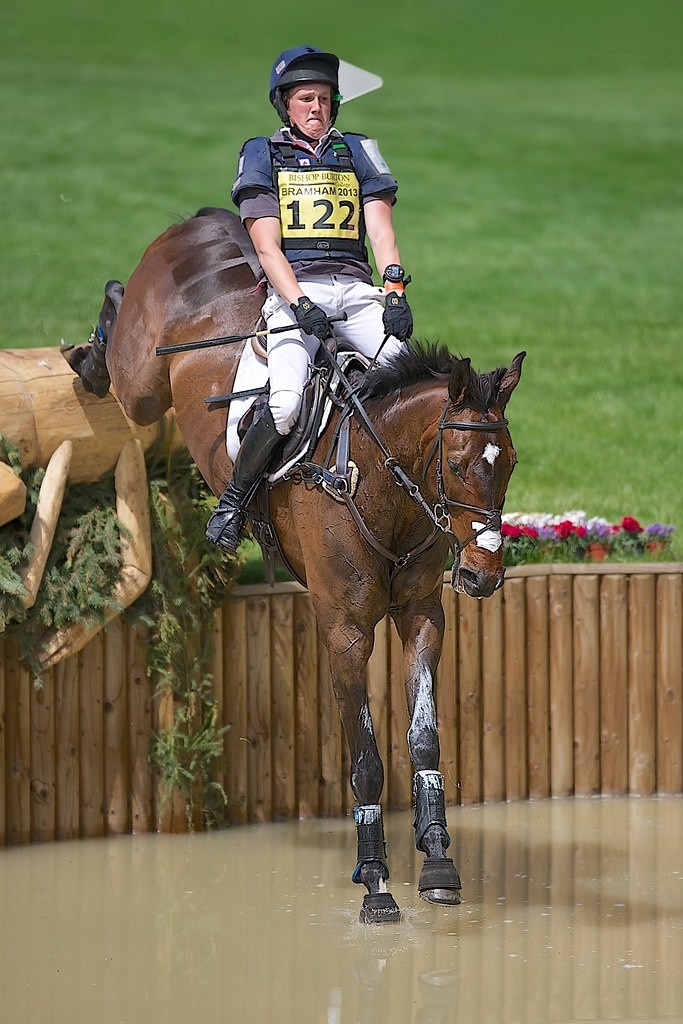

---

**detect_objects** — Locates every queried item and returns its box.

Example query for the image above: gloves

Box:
[289,296,331,338]
[382,291,414,342]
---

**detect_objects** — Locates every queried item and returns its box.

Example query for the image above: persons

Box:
[205,44,415,554]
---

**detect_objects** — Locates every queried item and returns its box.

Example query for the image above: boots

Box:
[205,402,291,553]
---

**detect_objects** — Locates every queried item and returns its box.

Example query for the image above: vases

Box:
[635,544,645,556]
[645,541,666,553]
[577,547,585,558]
[589,541,607,562]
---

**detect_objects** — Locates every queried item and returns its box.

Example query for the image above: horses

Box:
[65,203,527,934]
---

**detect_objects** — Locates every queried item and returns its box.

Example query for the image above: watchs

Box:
[382,264,412,289]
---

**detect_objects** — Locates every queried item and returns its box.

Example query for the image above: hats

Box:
[270,46,339,100]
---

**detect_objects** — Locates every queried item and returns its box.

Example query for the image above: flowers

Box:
[496,511,675,565]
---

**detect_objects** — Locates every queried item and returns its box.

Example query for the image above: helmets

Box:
[274,59,338,99]
